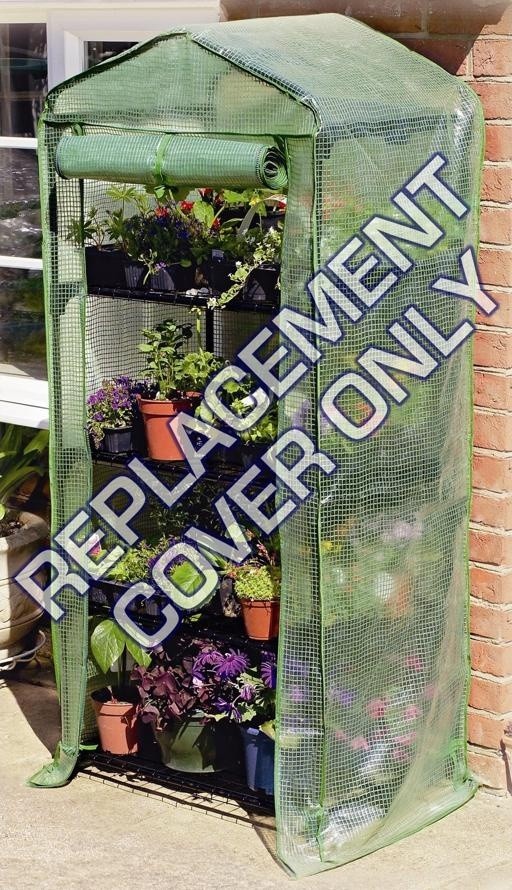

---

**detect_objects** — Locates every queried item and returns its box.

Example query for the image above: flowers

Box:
[68,178,435,765]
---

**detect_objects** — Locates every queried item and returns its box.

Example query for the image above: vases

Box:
[92,688,274,795]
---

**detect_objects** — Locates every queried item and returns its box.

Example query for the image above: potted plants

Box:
[0,423,51,672]
